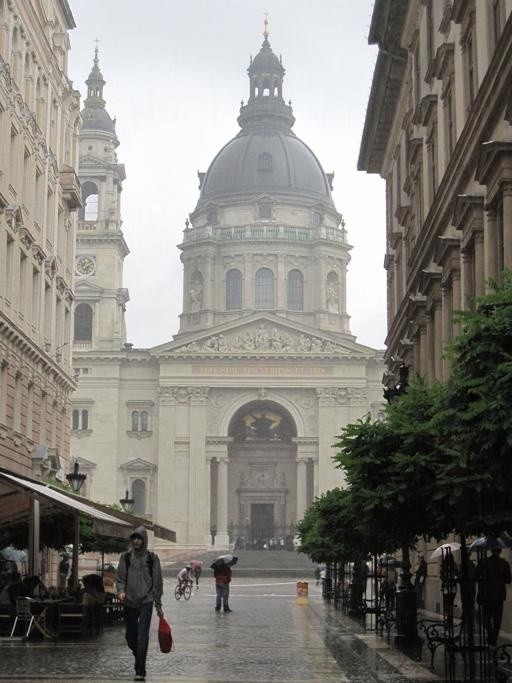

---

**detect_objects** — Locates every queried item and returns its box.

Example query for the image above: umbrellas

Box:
[470,537,512,551]
[318,563,327,570]
[189,560,203,567]
[209,555,238,569]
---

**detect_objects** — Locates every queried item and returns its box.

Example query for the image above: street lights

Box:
[382,363,418,634]
[65,460,87,601]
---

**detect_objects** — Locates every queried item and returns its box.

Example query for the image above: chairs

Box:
[9,592,122,639]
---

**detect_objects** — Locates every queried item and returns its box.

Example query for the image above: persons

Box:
[214,568,233,613]
[361,544,511,647]
[315,568,321,585]
[234,534,294,552]
[319,570,326,586]
[187,324,334,353]
[0,555,116,638]
[177,565,202,595]
[116,525,164,681]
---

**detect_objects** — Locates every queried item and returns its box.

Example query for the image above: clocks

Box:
[76,253,96,276]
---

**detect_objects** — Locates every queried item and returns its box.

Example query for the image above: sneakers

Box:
[215,607,232,612]
[135,675,144,680]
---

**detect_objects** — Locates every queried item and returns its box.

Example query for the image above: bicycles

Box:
[174,578,193,601]
